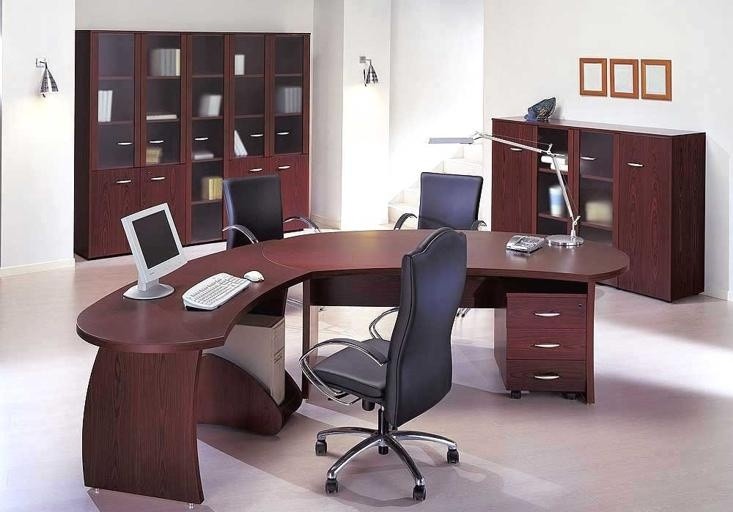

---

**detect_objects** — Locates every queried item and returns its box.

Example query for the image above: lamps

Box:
[426,129,586,247]
[359,57,379,89]
[34,57,58,98]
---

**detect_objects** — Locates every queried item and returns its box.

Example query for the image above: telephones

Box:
[506,235,545,253]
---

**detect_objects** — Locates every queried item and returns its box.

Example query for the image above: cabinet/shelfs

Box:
[74,29,311,262]
[492,278,587,400]
[490,116,706,303]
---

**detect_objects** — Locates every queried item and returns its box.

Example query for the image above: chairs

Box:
[297,226,468,502]
[219,174,324,313]
[393,171,489,318]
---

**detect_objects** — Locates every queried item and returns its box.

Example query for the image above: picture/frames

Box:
[578,57,672,100]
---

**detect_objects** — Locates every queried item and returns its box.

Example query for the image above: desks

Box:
[75,229,630,510]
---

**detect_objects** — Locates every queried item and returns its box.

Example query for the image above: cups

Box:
[548,186,565,217]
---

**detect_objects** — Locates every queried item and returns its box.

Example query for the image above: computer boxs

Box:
[201,313,285,407]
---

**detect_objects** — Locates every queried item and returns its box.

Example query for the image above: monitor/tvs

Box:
[120,202,187,300]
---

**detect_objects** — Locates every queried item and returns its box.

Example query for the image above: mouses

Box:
[243,270,265,282]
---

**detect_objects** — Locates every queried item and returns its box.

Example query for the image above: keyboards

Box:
[181,272,251,311]
